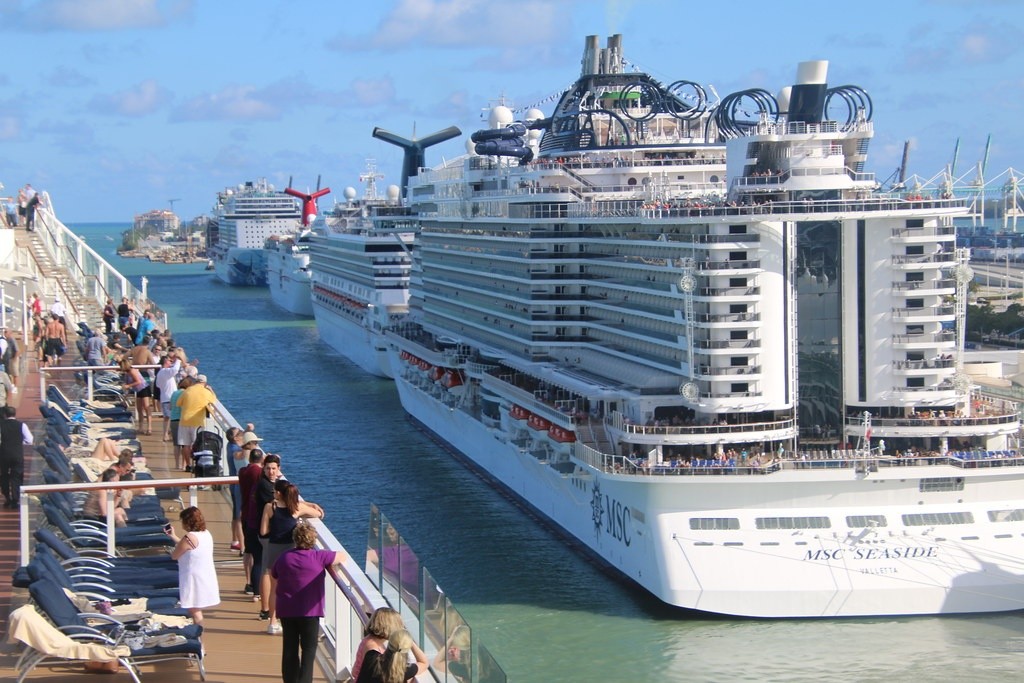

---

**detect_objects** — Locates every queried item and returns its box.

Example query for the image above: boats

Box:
[439,370,461,393]
[203,175,311,286]
[526,413,550,441]
[399,351,410,367]
[407,355,421,372]
[547,423,577,454]
[259,183,333,318]
[312,287,366,315]
[507,404,531,429]
[417,360,431,378]
[427,366,444,385]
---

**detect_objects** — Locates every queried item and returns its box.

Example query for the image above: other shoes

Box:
[268,624,283,634]
[230,542,241,551]
[186,465,194,471]
[146,431,151,435]
[164,437,174,442]
[137,429,146,434]
[245,584,254,595]
[4,498,20,508]
[252,595,261,601]
[259,609,271,620]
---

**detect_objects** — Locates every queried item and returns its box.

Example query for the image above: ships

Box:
[378,27,1023,623]
[306,124,462,381]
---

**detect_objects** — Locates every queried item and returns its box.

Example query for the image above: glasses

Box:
[155,347,161,351]
[234,432,241,439]
[126,469,129,473]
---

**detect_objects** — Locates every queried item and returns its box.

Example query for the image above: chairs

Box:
[619,414,1023,474]
[14,323,206,683]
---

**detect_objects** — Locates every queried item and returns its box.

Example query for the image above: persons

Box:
[620,411,728,432]
[164,506,222,658]
[893,446,1024,468]
[268,523,347,683]
[751,165,784,183]
[609,448,806,476]
[940,327,952,341]
[935,353,953,368]
[937,250,943,261]
[810,423,831,441]
[0,290,430,682]
[526,154,726,168]
[513,179,559,192]
[800,197,814,213]
[0,182,43,233]
[610,135,639,146]
[641,198,774,214]
[908,398,1015,425]
[943,300,950,313]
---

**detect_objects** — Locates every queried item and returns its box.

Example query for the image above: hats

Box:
[198,374,207,382]
[241,431,264,448]
